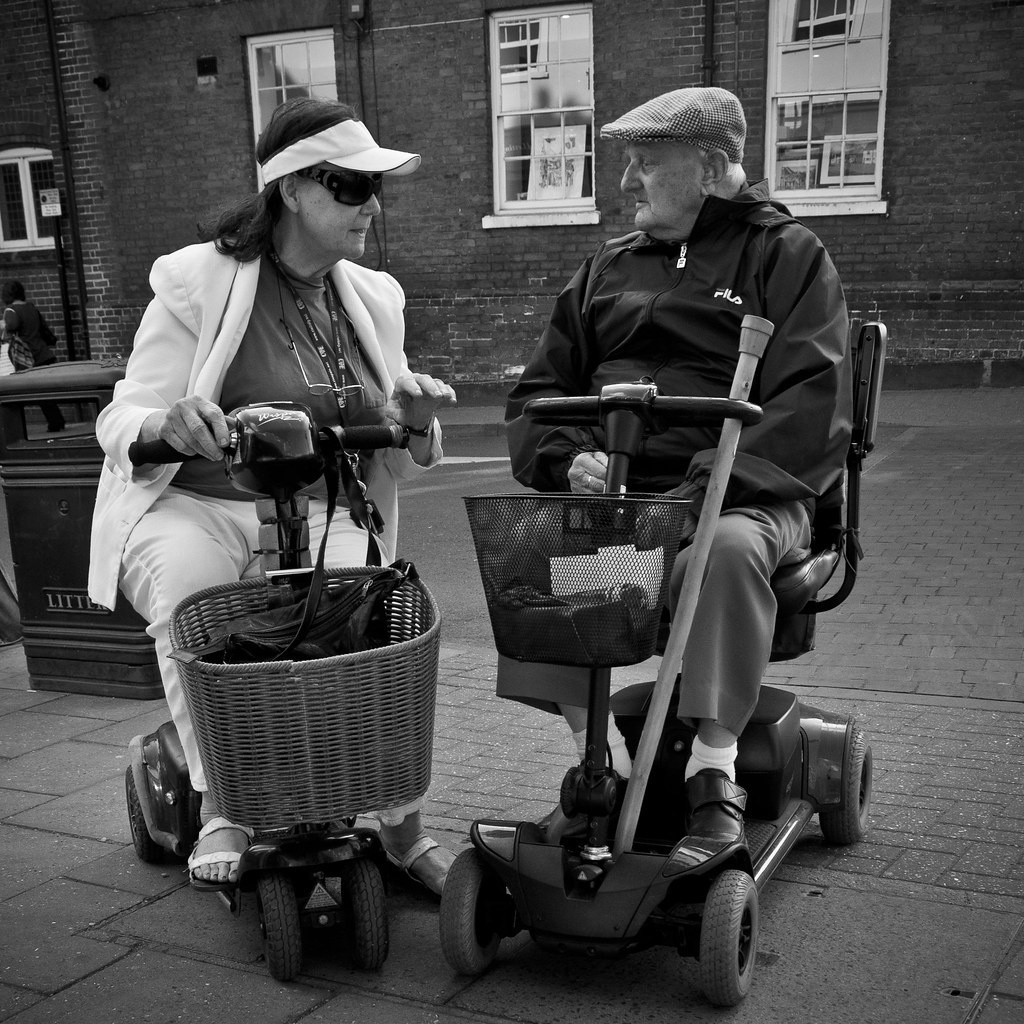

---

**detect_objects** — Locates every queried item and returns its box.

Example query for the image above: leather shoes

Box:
[681,768,747,850]
[538,800,591,842]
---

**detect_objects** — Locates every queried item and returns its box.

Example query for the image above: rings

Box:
[587,476,592,487]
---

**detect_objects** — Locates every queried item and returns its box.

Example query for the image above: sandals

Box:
[376,829,460,898]
[188,817,255,894]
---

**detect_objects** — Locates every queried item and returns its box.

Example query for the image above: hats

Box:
[600,87,747,163]
[260,118,421,185]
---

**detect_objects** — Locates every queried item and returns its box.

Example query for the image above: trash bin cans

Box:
[0,359,167,701]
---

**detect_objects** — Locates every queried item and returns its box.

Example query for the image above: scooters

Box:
[439,300,885,1007]
[125,383,441,982]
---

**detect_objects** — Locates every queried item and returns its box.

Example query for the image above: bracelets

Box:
[406,419,434,438]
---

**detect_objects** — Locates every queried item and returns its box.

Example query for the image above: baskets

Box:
[460,491,695,667]
[169,567,441,828]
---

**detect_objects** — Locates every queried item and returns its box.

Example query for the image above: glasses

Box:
[286,328,365,397]
[297,167,384,206]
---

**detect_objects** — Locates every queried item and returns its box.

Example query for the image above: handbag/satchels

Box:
[488,581,646,664]
[165,560,414,664]
[2,308,37,369]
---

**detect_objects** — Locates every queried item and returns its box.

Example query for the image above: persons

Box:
[495,86,856,877]
[0,560,22,647]
[87,97,459,896]
[0,280,67,431]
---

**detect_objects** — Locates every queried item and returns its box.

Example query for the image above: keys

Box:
[357,480,379,535]
